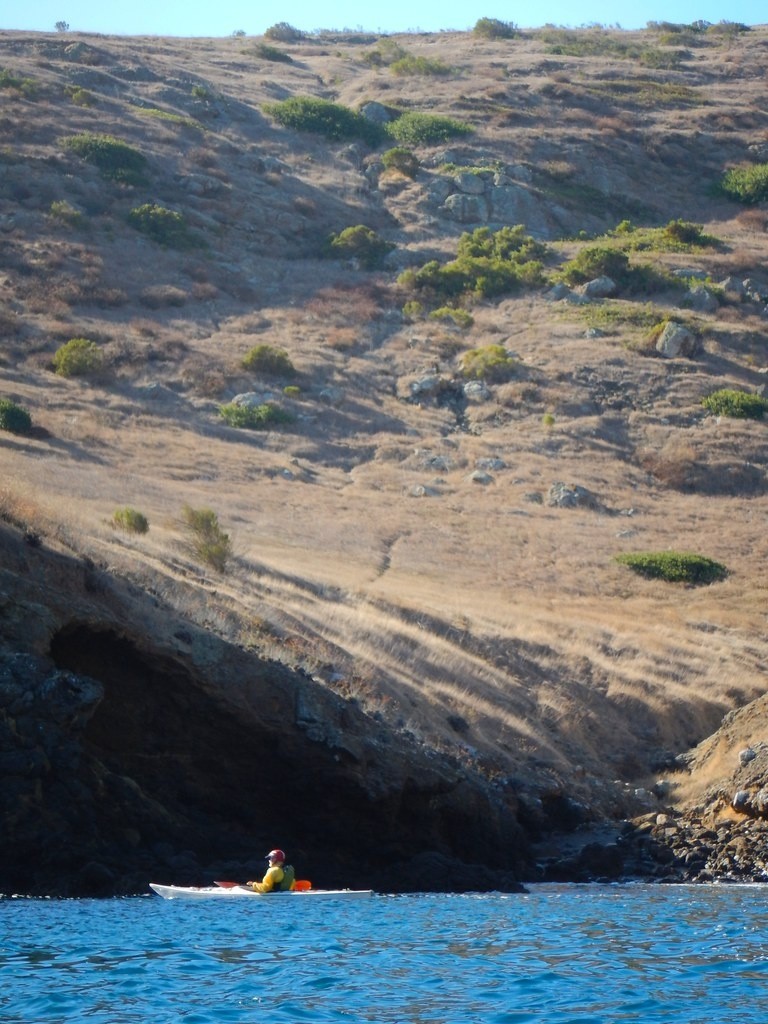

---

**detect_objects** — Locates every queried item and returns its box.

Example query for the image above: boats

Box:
[149,883,374,901]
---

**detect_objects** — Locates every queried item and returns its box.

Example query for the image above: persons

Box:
[247,850,295,894]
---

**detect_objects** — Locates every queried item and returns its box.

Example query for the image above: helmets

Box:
[265,850,285,862]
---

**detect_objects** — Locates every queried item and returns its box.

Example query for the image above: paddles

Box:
[214,881,312,890]
